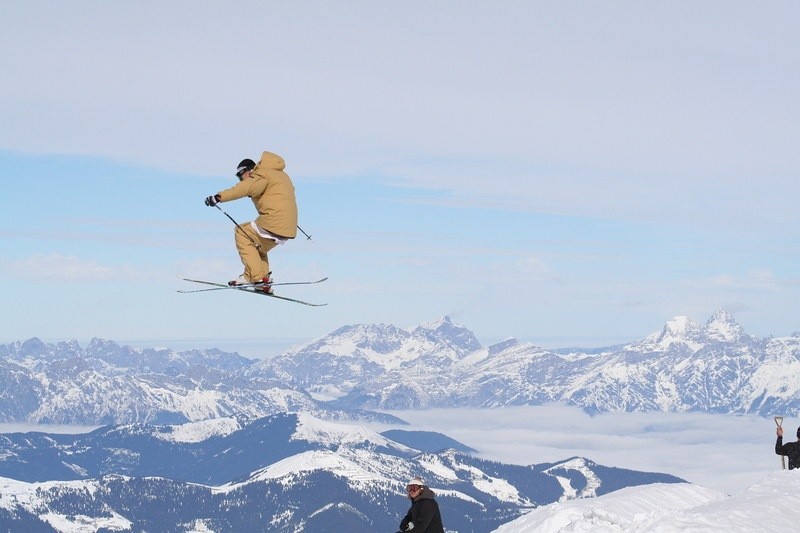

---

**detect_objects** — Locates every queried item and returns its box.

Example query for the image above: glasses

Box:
[407,484,419,492]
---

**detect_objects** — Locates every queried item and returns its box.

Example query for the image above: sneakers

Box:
[239,284,274,295]
[228,273,270,286]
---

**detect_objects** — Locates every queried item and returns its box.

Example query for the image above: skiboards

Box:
[177,278,326,306]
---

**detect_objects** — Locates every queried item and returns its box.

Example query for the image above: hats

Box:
[236,159,256,177]
[796,426,800,438]
[409,477,424,490]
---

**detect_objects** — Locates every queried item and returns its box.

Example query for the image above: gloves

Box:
[205,194,222,207]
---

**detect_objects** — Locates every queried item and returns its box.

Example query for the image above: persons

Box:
[775,426,800,470]
[205,150,298,295]
[395,476,445,533]
